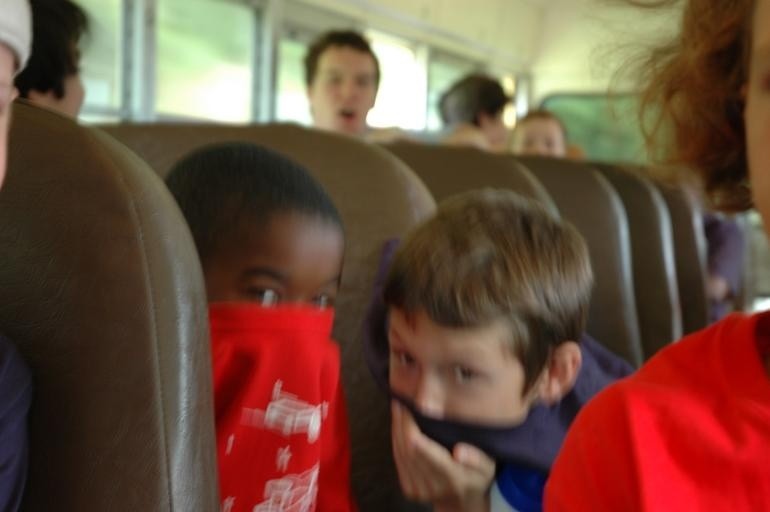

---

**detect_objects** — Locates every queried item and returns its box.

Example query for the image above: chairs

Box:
[0,102,754,509]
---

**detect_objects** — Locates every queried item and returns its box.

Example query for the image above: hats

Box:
[438,73,512,128]
